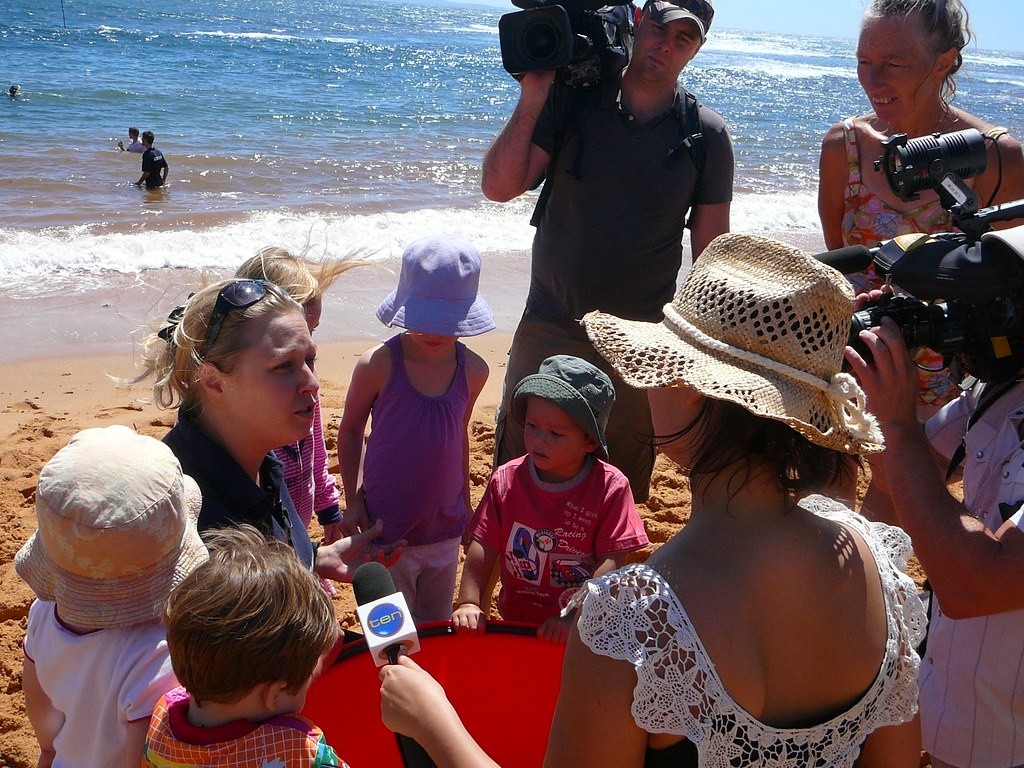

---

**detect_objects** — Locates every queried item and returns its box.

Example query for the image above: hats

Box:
[579,233,886,456]
[641,0,714,42]
[15,425,209,629]
[375,236,495,337]
[511,355,616,462]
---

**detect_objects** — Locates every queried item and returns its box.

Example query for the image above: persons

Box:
[234,244,344,598]
[817,0,1024,525]
[119,127,145,153]
[141,523,354,768]
[482,0,735,504]
[845,283,1024,768]
[337,236,497,624]
[139,279,408,584]
[454,355,649,644]
[12,425,210,768]
[136,130,170,188]
[381,232,929,768]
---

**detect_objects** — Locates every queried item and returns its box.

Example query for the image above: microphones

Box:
[814,243,882,275]
[352,562,437,768]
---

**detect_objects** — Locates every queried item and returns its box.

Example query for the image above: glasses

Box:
[198,279,291,359]
